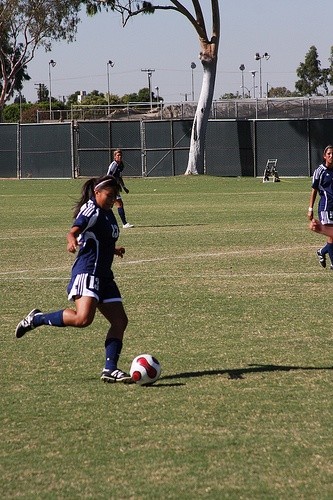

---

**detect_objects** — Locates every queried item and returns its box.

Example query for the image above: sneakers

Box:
[123,222,134,228]
[101,367,133,383]
[317,249,327,268]
[15,308,41,338]
[330,264,333,269]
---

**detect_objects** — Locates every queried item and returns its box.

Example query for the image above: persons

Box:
[105,149,134,229]
[307,145,333,269]
[14,175,131,383]
[309,218,332,236]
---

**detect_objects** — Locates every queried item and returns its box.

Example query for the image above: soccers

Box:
[130,355,160,386]
